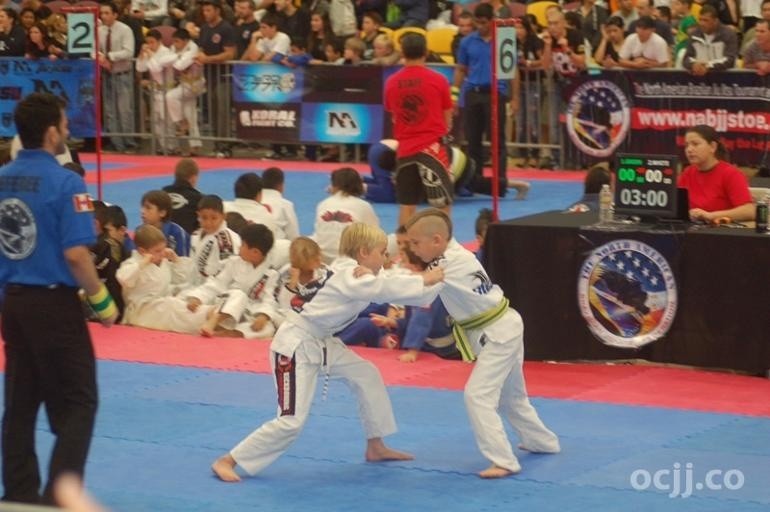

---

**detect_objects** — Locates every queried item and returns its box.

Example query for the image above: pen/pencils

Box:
[732,221,747,227]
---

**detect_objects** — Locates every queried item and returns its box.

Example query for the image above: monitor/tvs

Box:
[615,153,678,218]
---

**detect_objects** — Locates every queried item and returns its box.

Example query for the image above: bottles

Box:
[756,200,769,230]
[599,184,614,230]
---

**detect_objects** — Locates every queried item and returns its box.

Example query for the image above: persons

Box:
[546,5,561,17]
[25,23,57,61]
[738,1,770,59]
[223,172,293,270]
[129,0,168,27]
[683,5,738,75]
[740,0,769,33]
[226,212,248,234]
[305,8,345,61]
[675,124,758,223]
[452,3,520,190]
[212,222,444,484]
[360,11,386,57]
[328,0,357,46]
[142,189,191,257]
[619,15,669,70]
[114,224,223,336]
[136,28,176,156]
[385,226,408,315]
[441,143,531,199]
[526,13,545,40]
[273,237,388,348]
[675,0,700,37]
[342,37,371,65]
[313,168,381,265]
[48,13,68,60]
[565,12,582,29]
[611,0,639,33]
[706,1,739,31]
[541,10,585,170]
[406,208,560,477]
[397,32,446,63]
[690,0,705,25]
[262,37,312,68]
[740,21,770,76]
[163,160,203,234]
[371,33,400,66]
[103,204,137,261]
[259,168,301,240]
[0,92,118,507]
[594,15,634,68]
[384,32,452,223]
[232,0,260,59]
[13,7,36,58]
[239,13,291,61]
[652,5,688,68]
[90,3,141,152]
[64,163,86,177]
[450,12,476,64]
[325,138,400,204]
[472,207,497,262]
[36,5,53,24]
[572,0,609,52]
[185,224,280,340]
[271,0,315,57]
[384,1,430,31]
[496,5,512,18]
[515,15,548,169]
[628,0,675,45]
[196,1,239,63]
[160,27,206,157]
[79,199,124,324]
[163,0,197,28]
[0,7,26,57]
[190,195,242,285]
[369,245,463,362]
[114,1,144,59]
[309,38,346,66]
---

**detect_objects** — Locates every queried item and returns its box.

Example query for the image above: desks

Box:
[488,209,770,379]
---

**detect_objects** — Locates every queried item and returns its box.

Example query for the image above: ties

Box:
[106,29,111,54]
